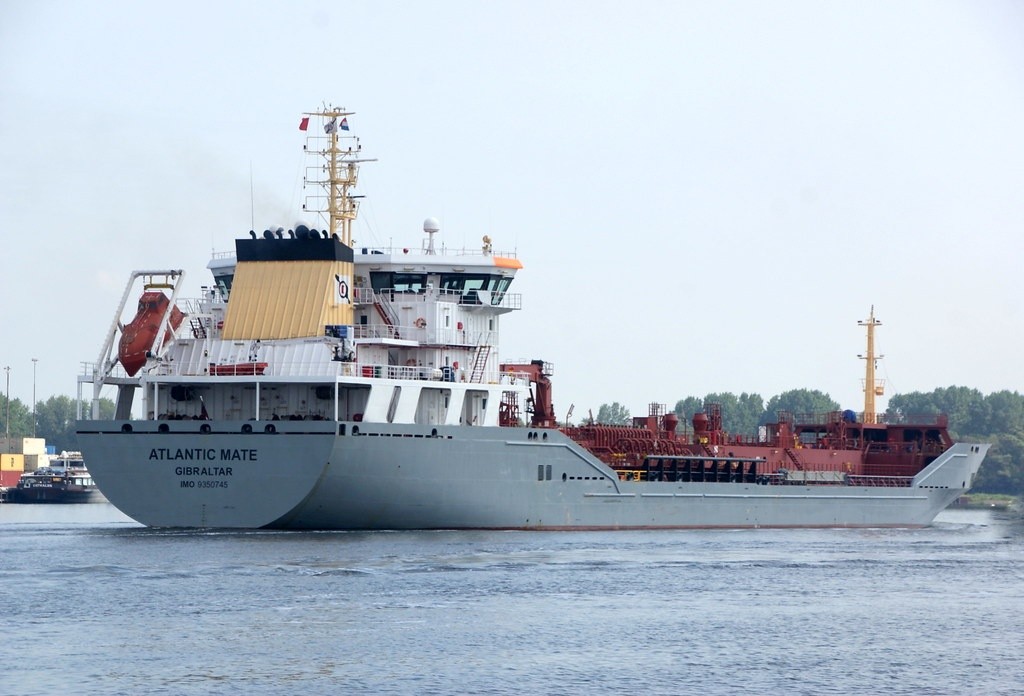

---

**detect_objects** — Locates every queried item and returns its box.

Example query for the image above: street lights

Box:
[4,366,11,435]
[31,358,38,437]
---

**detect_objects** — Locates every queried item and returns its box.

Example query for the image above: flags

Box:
[324,118,337,134]
[340,117,349,132]
[299,117,309,131]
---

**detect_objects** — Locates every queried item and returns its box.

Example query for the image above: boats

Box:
[1,466,111,504]
[72,101,993,534]
